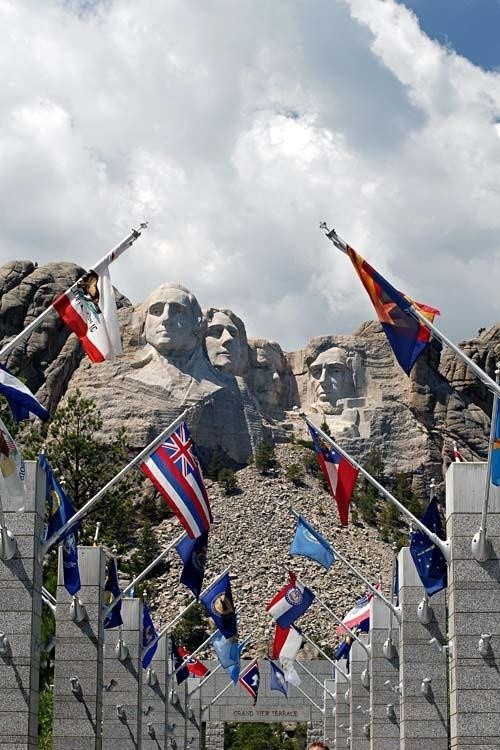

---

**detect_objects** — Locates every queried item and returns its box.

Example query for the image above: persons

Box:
[306,741,328,750]
